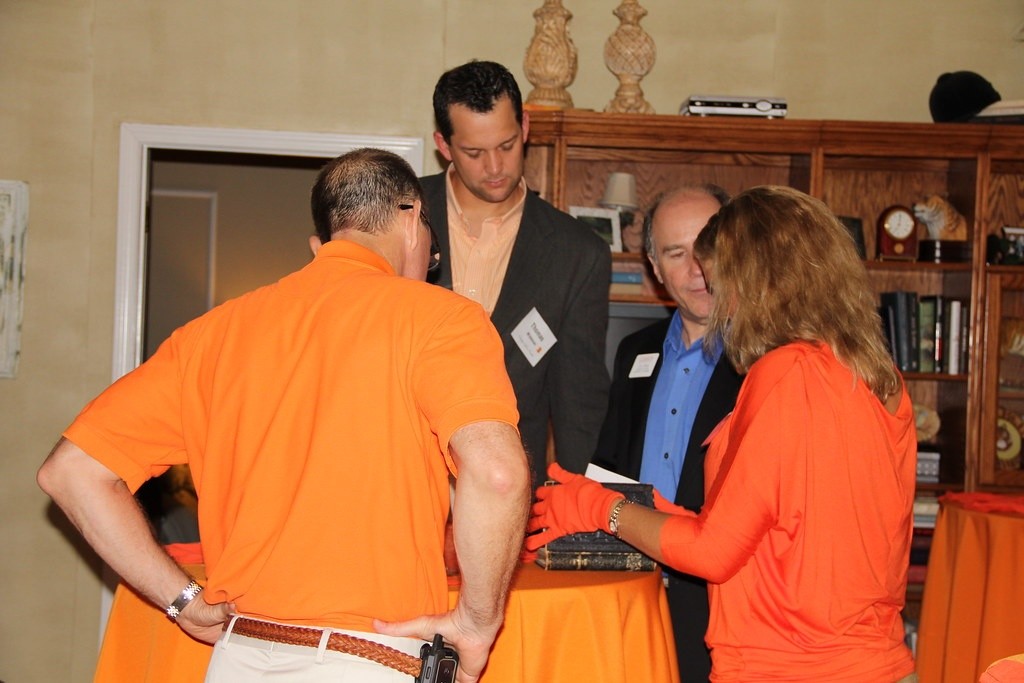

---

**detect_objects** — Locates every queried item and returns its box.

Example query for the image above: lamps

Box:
[600,173,637,212]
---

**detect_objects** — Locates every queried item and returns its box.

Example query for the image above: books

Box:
[534,481,659,573]
[874,290,969,375]
[905,563,929,594]
[608,250,674,303]
[910,493,943,537]
[915,448,942,484]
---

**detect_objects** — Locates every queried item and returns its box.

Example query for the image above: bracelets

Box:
[165,581,203,620]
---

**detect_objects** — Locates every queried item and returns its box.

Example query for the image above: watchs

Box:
[608,499,635,540]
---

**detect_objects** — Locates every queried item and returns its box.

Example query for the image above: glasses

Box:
[398,204,442,272]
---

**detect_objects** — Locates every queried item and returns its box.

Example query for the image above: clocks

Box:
[879,206,918,264]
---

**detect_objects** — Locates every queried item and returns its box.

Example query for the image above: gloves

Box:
[525,463,626,550]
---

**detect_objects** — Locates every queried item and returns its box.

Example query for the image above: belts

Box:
[222,614,423,677]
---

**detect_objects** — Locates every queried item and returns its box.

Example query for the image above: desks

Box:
[915,489,1024,683]
[91,545,681,683]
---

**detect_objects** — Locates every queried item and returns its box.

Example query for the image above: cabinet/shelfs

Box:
[523,109,1024,594]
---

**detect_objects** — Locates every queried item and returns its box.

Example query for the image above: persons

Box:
[590,183,749,683]
[416,61,612,503]
[523,187,918,683]
[36,147,531,683]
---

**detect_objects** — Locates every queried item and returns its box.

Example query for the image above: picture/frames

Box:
[568,206,623,253]
